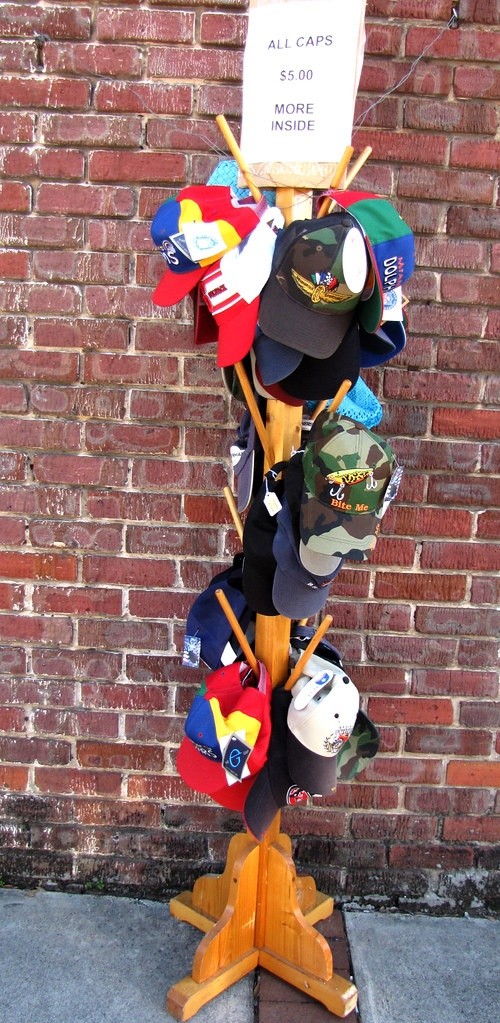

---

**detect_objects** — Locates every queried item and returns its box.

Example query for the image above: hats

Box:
[176,553,380,843]
[230,404,404,621]
[149,157,417,431]
